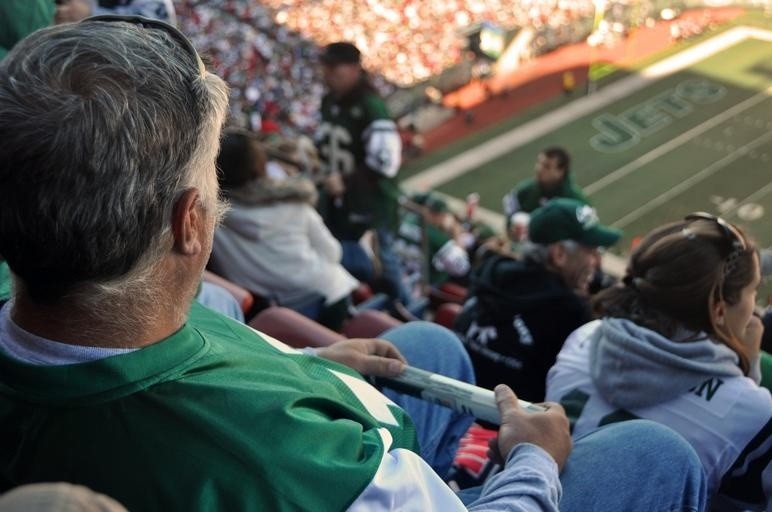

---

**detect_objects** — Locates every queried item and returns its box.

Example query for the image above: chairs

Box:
[194,260,526,401]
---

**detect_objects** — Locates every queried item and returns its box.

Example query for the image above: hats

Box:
[528,196,625,250]
[316,40,362,68]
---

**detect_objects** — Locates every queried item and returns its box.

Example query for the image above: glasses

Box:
[72,10,209,87]
[683,210,748,258]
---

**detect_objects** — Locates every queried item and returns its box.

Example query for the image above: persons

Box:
[3,0,771,512]
